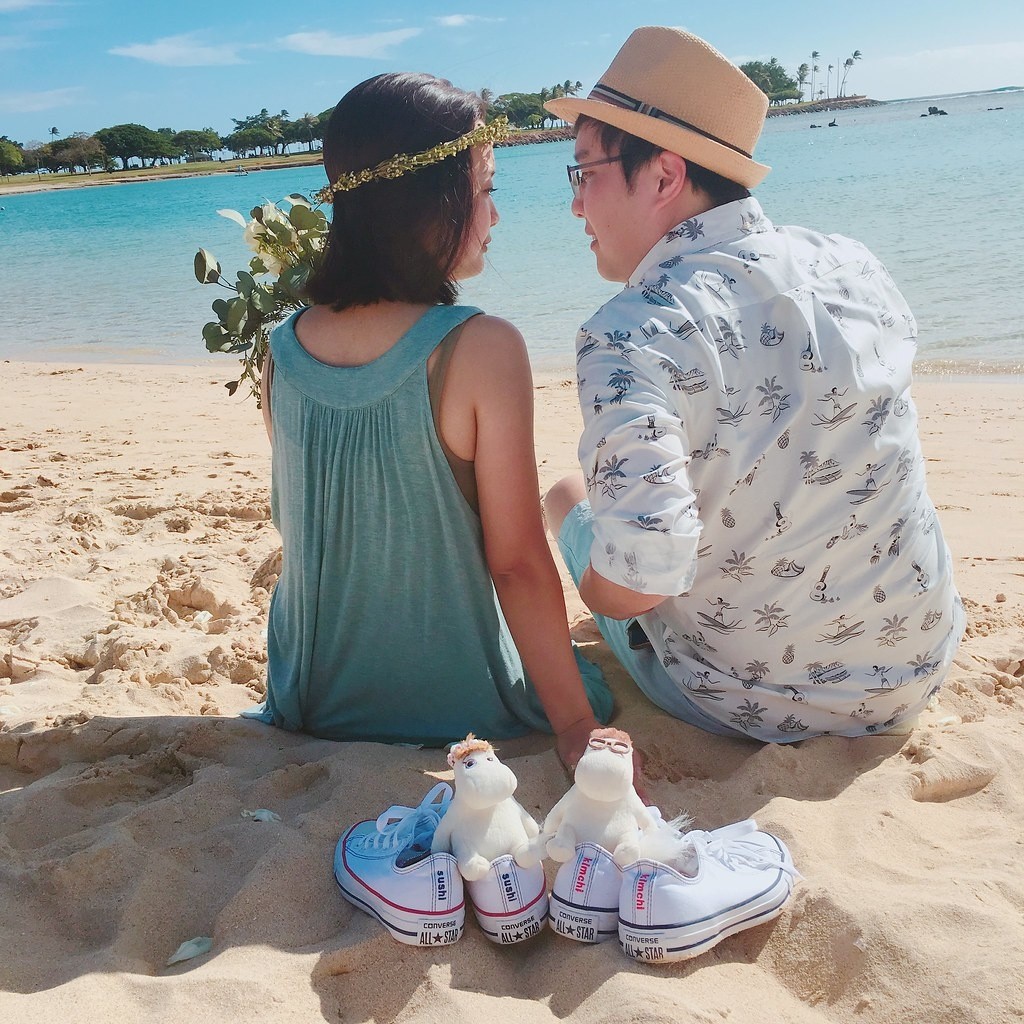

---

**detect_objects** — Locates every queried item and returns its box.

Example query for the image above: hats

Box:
[543,25,773,190]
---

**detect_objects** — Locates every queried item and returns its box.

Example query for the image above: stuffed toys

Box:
[430,734,547,882]
[544,728,684,865]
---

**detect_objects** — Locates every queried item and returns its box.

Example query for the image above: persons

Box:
[238,165,242,174]
[545,27,966,746]
[257,71,649,806]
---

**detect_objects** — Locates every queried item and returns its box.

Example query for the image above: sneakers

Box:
[553,821,796,966]
[334,782,550,946]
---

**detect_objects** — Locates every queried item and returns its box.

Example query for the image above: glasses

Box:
[566,154,631,200]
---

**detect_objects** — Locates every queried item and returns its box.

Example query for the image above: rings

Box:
[568,765,575,774]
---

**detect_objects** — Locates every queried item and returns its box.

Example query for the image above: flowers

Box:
[193,193,332,409]
